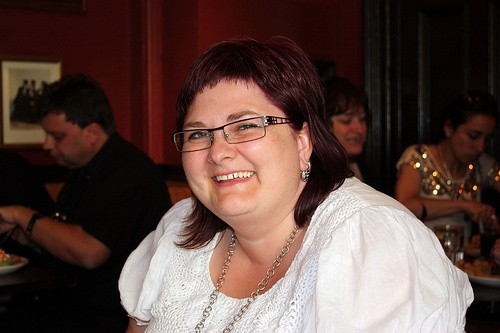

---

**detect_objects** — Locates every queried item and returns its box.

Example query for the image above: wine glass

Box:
[434,223,465,266]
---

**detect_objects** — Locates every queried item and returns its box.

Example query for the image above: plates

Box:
[458,260,500,286]
[0,257,29,274]
[465,249,481,257]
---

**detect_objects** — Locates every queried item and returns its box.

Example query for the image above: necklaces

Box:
[195,225,299,333]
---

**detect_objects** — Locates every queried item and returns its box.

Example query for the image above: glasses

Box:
[171,113,289,153]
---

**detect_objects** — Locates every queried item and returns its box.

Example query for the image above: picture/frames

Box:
[0,55,65,149]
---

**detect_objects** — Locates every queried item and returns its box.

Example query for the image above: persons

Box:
[325,78,398,198]
[395,82,500,265]
[118,34,473,333]
[0,73,174,333]
[9,80,48,123]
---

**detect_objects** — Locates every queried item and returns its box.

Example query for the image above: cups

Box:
[479,214,497,260]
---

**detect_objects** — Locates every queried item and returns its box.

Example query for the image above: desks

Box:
[447,251,500,333]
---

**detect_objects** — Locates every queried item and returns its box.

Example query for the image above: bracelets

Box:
[26,211,47,240]
